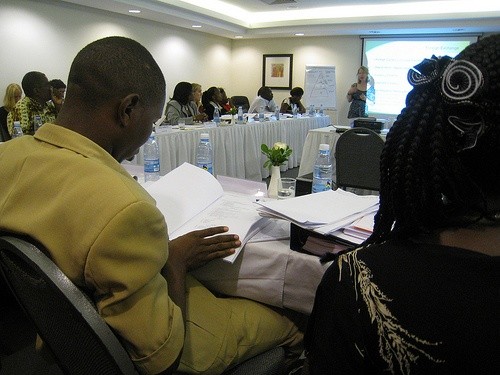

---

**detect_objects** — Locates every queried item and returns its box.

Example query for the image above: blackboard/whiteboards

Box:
[303,65,337,111]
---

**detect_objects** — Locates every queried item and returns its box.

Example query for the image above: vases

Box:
[267,166,283,199]
[230,115,235,125]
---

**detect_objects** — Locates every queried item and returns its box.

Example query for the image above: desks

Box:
[308,125,390,183]
[122,111,327,182]
[119,164,381,316]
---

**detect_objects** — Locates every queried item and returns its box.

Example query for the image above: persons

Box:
[0,34,305,375]
[297,29,499,375]
[6,70,57,138]
[279,86,306,114]
[248,86,280,113]
[190,82,207,121]
[0,83,23,142]
[218,88,236,112]
[201,87,228,120]
[49,79,66,107]
[159,81,208,126]
[346,65,377,118]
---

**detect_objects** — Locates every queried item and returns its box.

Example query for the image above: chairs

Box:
[335,126,386,196]
[0,228,138,375]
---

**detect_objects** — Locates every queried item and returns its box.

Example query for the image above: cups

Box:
[278,178,296,198]
[178,120,185,130]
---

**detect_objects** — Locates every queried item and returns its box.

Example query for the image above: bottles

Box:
[309,105,315,117]
[237,106,243,124]
[312,144,332,193]
[12,121,23,139]
[34,115,42,133]
[293,104,297,119]
[214,107,220,126]
[319,104,323,116]
[195,133,212,175]
[144,132,160,182]
[275,106,279,120]
[259,106,264,122]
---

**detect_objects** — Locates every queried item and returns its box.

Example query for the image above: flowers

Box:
[260,141,292,170]
[225,104,238,115]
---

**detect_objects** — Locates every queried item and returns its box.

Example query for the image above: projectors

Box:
[354,118,386,130]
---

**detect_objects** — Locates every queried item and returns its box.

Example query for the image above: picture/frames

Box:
[262,54,294,90]
